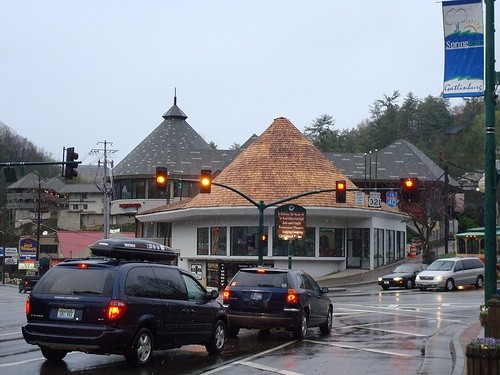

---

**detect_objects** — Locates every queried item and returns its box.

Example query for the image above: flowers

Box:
[467,336,500,349]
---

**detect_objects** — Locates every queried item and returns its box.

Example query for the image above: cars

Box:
[222,265,334,339]
[19,276,40,294]
[377,264,430,290]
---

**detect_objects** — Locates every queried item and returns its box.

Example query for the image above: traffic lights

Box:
[155,167,168,193]
[335,180,346,203]
[200,169,213,194]
[65,147,79,180]
[398,177,418,194]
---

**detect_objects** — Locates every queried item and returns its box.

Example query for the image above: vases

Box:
[466,347,500,375]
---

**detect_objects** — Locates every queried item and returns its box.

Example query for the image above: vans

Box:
[415,257,486,291]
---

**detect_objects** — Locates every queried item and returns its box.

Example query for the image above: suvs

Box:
[22,237,230,366]
